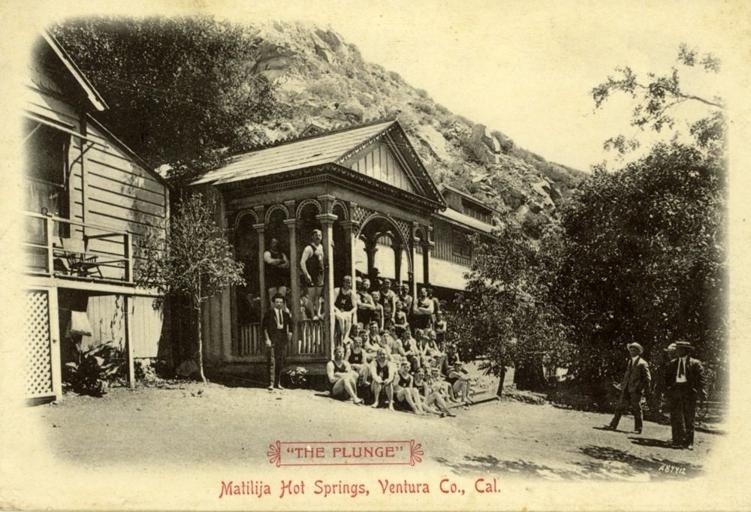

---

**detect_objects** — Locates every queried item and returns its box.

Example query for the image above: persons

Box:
[604,341,651,434]
[655,340,706,450]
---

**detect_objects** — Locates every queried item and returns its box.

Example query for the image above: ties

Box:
[629,358,632,373]
[278,309,282,325]
[678,358,684,378]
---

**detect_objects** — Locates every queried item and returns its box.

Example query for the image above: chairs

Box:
[61,236,102,277]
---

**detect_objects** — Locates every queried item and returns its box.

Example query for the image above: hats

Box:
[672,341,694,351]
[627,342,643,355]
[272,293,285,303]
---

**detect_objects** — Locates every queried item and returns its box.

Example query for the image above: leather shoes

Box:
[604,425,615,430]
[631,430,640,434]
[268,385,283,390]
[668,442,694,450]
